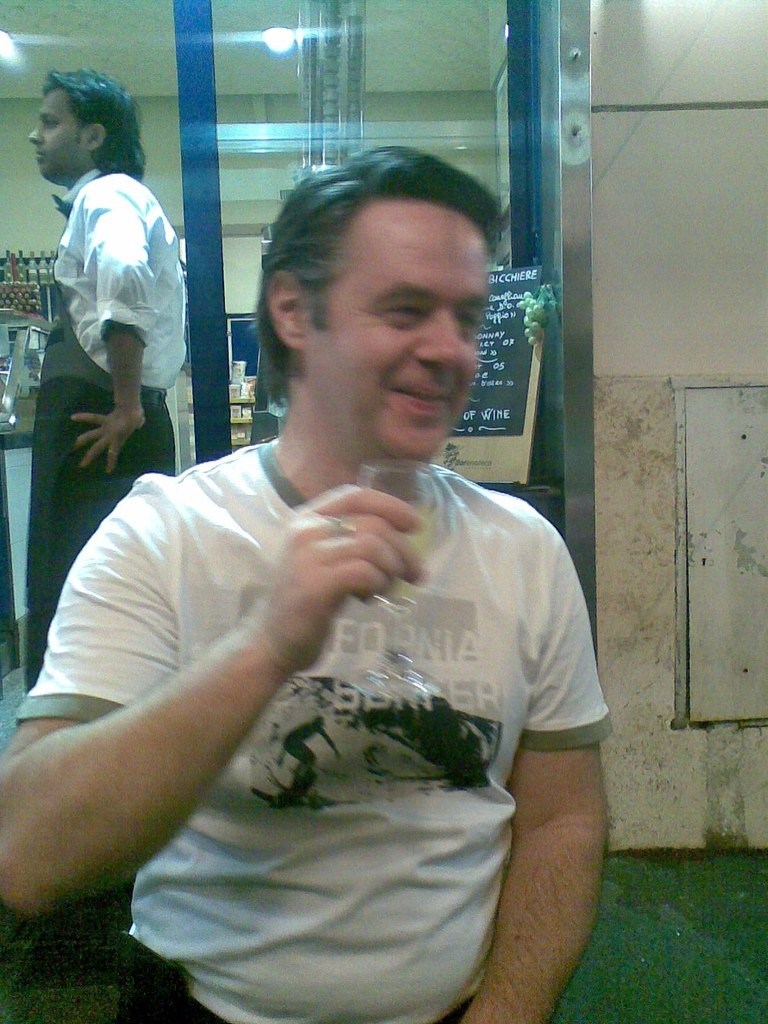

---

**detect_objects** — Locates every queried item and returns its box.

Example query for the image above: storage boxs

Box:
[230,362,257,440]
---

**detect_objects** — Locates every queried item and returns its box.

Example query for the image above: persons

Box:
[0,145,611,1024]
[25,67,187,695]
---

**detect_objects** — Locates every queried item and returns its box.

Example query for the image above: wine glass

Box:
[356,458,444,702]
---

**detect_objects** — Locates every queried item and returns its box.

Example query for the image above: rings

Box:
[324,514,358,533]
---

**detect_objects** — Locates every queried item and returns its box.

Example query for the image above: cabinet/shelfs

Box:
[230,399,254,445]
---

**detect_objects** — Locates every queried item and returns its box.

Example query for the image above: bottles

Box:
[4,250,56,285]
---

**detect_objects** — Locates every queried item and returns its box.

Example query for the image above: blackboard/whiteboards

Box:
[446,263,551,437]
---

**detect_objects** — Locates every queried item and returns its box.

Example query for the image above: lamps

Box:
[261,26,296,54]
